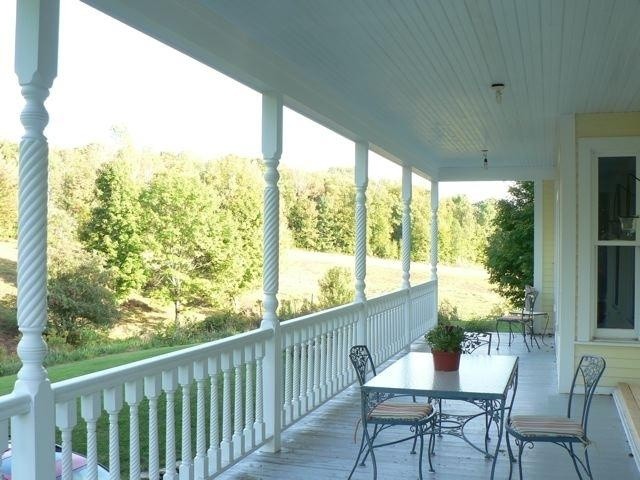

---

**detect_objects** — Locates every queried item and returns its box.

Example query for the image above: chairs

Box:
[505,354,606,480]
[348,345,436,479]
[495,291,540,352]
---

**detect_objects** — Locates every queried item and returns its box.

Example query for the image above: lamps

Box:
[619,173,639,238]
[607,182,634,236]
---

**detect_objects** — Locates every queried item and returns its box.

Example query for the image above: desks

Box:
[361,351,519,479]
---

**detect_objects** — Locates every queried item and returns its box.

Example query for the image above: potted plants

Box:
[425,319,464,371]
